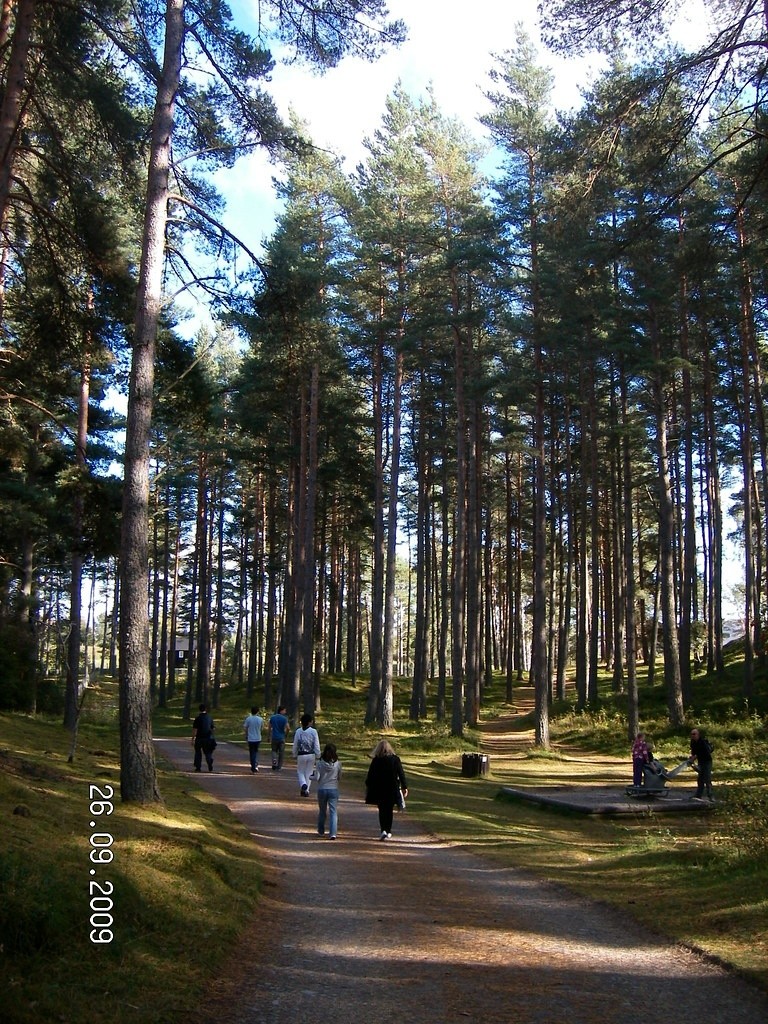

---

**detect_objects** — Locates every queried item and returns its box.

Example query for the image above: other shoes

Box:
[272,766,282,770]
[379,831,387,840]
[210,766,214,771]
[251,766,259,772]
[326,835,336,839]
[195,768,202,772]
[634,784,641,788]
[387,832,392,838]
[301,784,310,797]
[318,832,325,837]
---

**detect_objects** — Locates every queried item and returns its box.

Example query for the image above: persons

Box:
[192,704,218,773]
[364,740,408,841]
[631,732,649,788]
[646,742,658,772]
[268,706,290,770]
[309,743,343,840]
[292,714,321,797]
[244,705,264,774]
[689,729,716,802]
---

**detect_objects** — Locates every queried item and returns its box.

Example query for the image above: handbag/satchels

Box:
[398,787,406,810]
